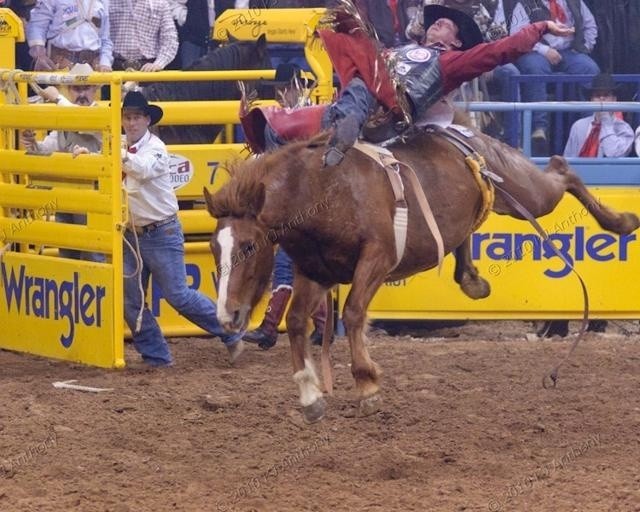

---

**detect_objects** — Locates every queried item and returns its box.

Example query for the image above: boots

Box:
[242,285,292,351]
[309,296,336,346]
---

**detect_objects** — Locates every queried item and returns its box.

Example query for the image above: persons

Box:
[241,63,336,351]
[36,85,244,369]
[19,62,107,264]
[24,0,640,166]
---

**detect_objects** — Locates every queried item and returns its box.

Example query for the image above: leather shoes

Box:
[321,111,362,168]
[126,359,176,373]
[227,340,244,365]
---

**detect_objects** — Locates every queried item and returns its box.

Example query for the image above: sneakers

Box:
[532,128,546,144]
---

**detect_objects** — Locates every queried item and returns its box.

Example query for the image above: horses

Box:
[142,27,277,209]
[202,112,640,425]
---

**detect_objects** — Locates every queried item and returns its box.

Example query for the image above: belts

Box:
[113,57,156,64]
[126,214,178,234]
[52,46,101,60]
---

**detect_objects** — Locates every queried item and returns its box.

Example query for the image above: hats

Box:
[120,91,163,126]
[66,63,94,73]
[260,62,307,88]
[581,72,633,102]
[423,4,484,50]
